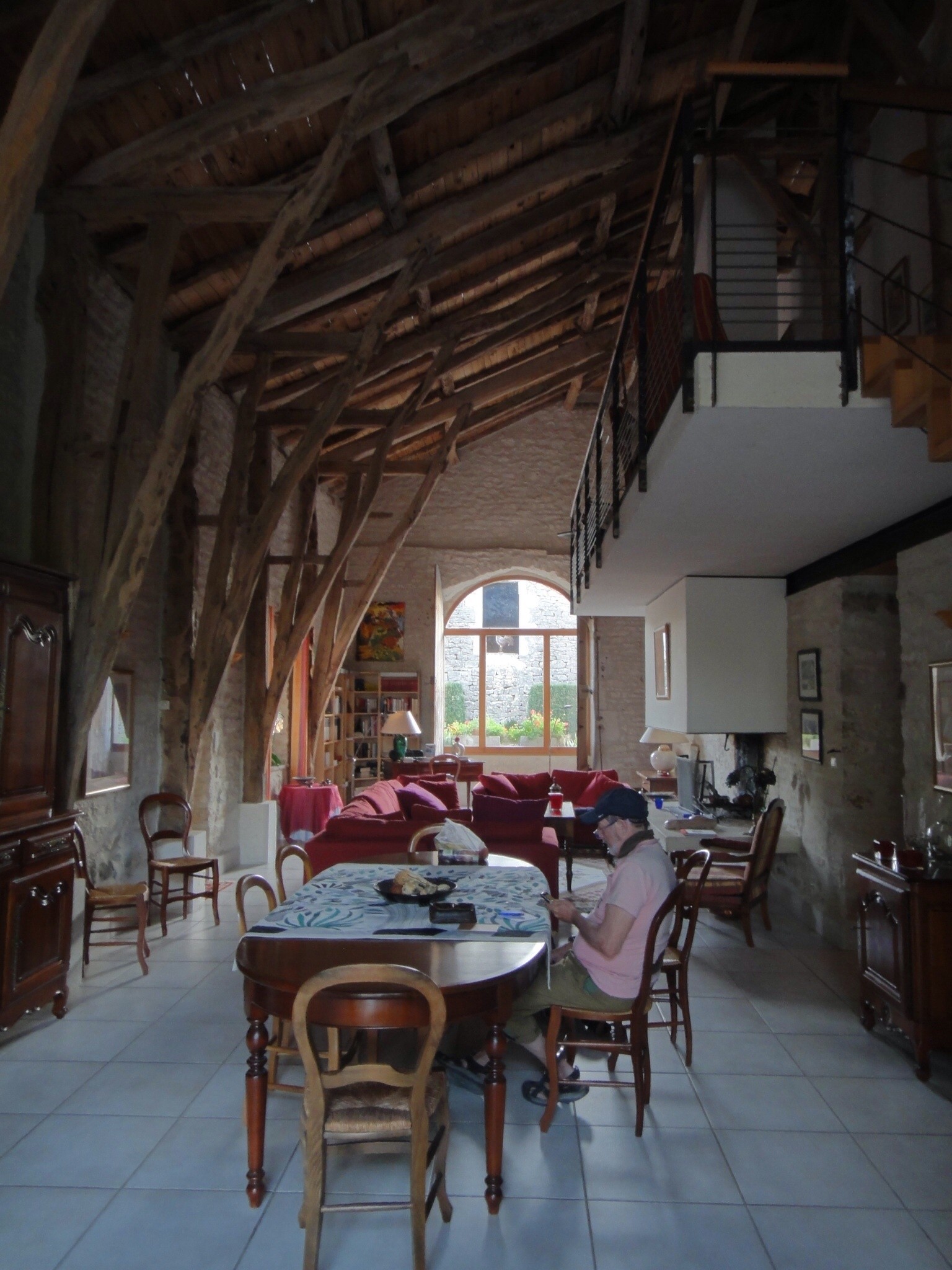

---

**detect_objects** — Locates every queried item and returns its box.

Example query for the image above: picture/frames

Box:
[880,255,913,335]
[796,648,823,701]
[917,281,934,338]
[928,660,952,787]
[799,708,824,766]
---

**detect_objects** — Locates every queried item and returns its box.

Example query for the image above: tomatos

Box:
[391,883,403,894]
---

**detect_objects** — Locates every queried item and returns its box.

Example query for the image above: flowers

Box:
[448,716,503,735]
[519,709,568,737]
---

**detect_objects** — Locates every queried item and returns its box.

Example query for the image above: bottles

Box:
[549,776,561,793]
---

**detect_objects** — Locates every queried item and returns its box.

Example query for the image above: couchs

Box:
[471,769,632,839]
[304,772,559,931]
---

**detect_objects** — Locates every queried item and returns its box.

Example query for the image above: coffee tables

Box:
[544,800,576,892]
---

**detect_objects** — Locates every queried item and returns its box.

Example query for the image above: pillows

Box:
[470,790,550,840]
[394,782,447,820]
[576,772,622,806]
[477,773,519,800]
[416,779,459,809]
[411,803,472,821]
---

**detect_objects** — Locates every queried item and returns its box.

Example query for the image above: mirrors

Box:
[653,623,670,700]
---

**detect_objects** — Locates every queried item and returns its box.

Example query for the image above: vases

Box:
[520,736,558,746]
[455,735,501,746]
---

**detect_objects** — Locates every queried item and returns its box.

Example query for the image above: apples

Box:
[479,846,488,859]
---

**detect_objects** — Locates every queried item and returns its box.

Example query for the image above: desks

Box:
[278,783,344,844]
[384,759,485,781]
[645,797,754,874]
[228,854,551,1214]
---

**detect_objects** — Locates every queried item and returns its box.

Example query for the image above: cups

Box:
[655,798,662,809]
[548,793,564,813]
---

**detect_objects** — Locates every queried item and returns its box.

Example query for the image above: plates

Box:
[372,877,458,904]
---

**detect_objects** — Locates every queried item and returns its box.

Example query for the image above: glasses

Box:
[593,820,617,840]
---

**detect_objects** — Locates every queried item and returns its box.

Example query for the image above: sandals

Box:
[522,1066,589,1107]
[433,1051,485,1096]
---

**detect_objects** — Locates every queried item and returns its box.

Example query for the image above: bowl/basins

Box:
[292,777,316,784]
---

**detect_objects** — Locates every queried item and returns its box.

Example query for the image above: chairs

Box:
[683,798,787,948]
[298,964,454,1270]
[73,822,150,976]
[608,849,712,1072]
[428,754,461,782]
[139,794,220,937]
[276,843,313,901]
[237,874,377,1099]
[539,879,687,1137]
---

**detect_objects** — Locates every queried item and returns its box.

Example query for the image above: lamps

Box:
[639,727,690,776]
[381,710,422,756]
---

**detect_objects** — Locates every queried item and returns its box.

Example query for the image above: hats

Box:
[579,787,649,826]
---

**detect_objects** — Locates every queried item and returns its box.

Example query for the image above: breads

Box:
[395,869,451,895]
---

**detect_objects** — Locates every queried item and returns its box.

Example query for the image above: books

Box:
[324,679,419,795]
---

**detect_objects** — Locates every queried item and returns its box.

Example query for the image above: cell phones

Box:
[541,891,555,903]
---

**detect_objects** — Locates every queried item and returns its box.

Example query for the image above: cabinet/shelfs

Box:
[851,850,952,1082]
[0,555,86,1029]
[314,658,420,803]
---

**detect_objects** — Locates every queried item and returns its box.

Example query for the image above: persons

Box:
[444,786,677,1107]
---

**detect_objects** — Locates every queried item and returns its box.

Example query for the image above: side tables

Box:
[636,771,677,793]
